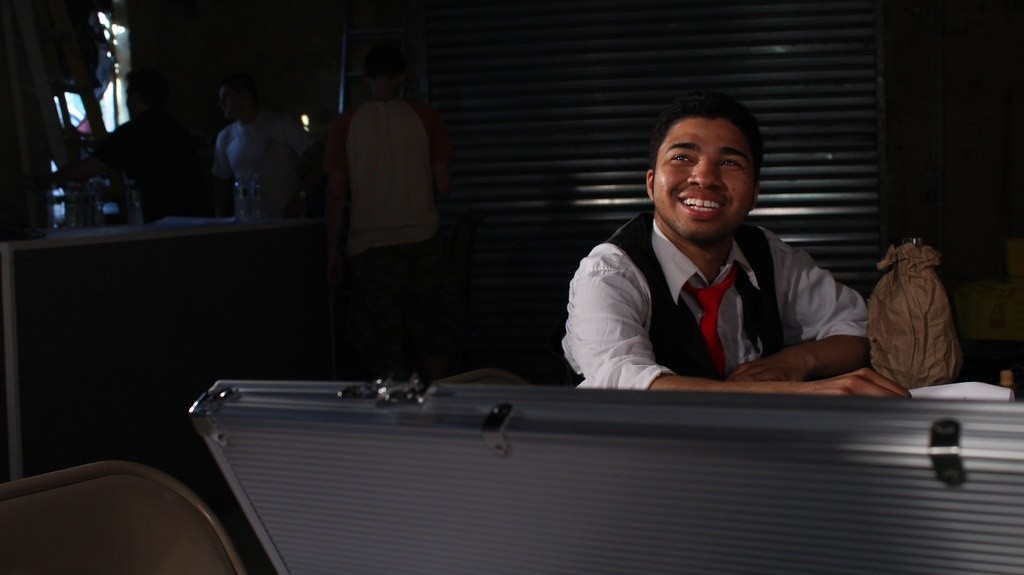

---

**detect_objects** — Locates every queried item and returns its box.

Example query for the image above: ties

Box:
[684,261,738,379]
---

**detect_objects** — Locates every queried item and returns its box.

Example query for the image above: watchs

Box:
[297,189,311,202]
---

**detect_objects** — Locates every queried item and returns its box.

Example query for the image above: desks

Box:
[0,216,338,482]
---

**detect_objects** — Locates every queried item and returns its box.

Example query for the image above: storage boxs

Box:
[954,236,1024,340]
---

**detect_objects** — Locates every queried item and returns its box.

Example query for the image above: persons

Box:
[562,91,912,399]
[69,67,210,223]
[211,74,325,221]
[322,45,453,380]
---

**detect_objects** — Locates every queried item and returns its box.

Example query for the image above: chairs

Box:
[0,460,251,574]
